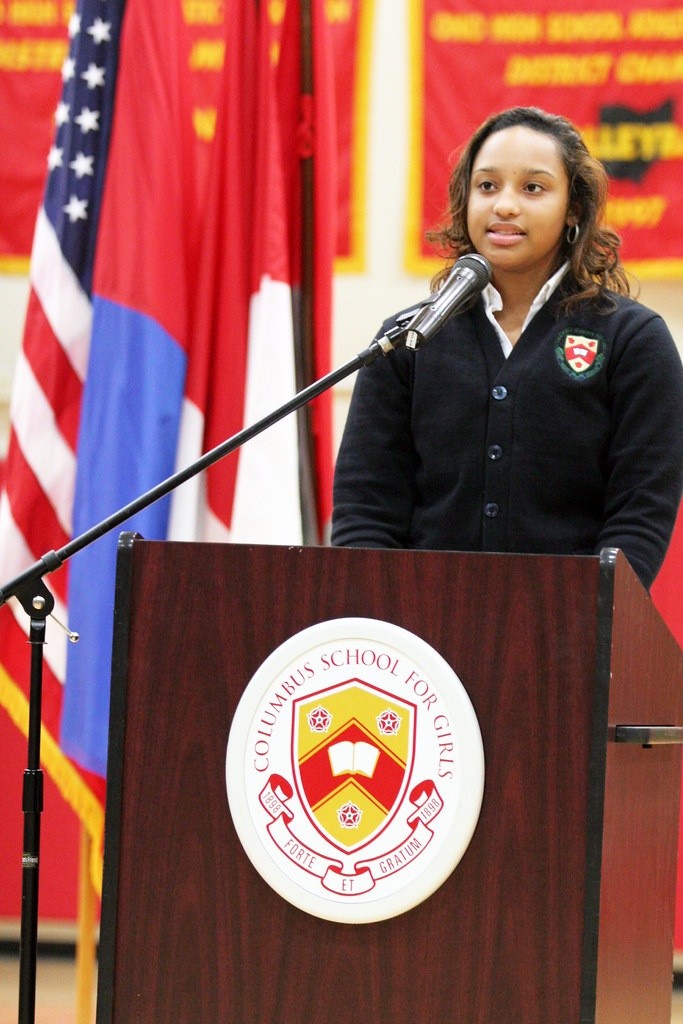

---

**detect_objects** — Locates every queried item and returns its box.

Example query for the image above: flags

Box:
[0,1,342,921]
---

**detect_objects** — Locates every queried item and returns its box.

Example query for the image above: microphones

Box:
[403,253,493,352]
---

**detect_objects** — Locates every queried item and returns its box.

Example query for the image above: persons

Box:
[328,105,683,596]
